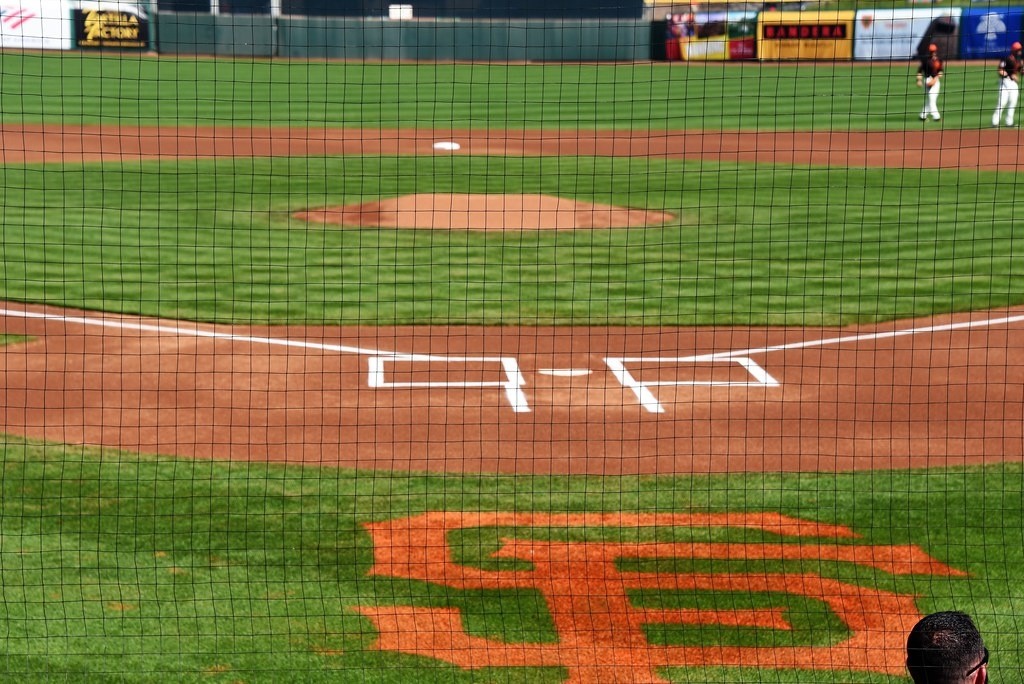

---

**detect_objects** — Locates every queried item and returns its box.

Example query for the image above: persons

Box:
[992,42,1024,129]
[915,45,944,122]
[905,610,991,684]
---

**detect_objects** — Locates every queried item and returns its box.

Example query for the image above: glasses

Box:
[966,647,989,677]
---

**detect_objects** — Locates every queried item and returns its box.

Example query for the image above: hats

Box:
[1011,42,1022,50]
[928,44,937,51]
